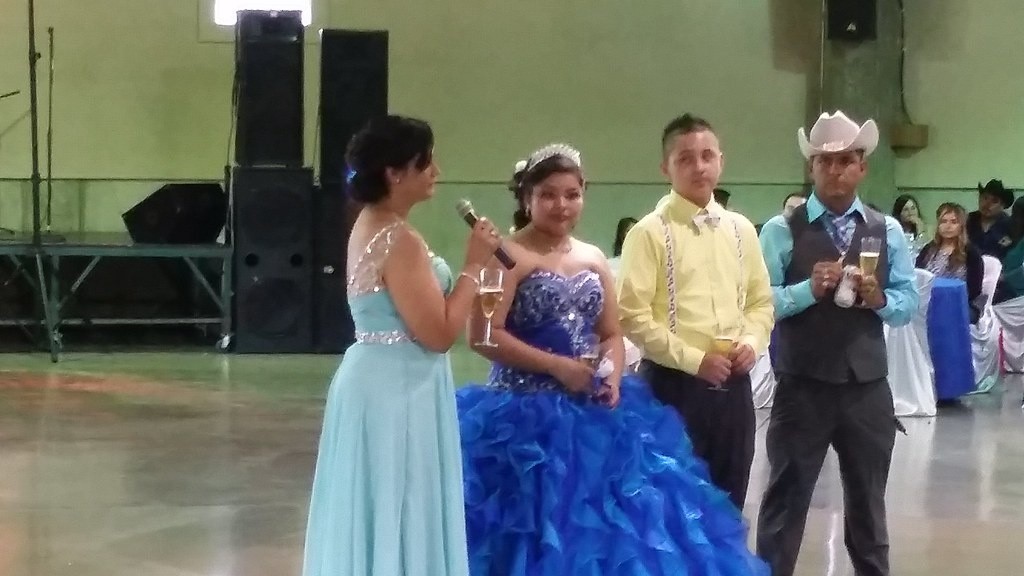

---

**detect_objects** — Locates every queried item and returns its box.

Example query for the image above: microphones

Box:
[455,199,517,271]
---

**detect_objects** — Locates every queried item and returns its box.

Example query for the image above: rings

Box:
[823,281,828,287]
[870,287,875,293]
[824,267,828,273]
[491,231,498,237]
[824,274,830,279]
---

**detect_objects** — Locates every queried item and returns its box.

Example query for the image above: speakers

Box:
[825,0,880,42]
[225,9,390,355]
[122,183,225,244]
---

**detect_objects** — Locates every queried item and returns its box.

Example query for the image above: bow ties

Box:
[691,211,721,237]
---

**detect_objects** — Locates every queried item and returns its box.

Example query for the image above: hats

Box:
[797,109,880,159]
[978,178,1014,209]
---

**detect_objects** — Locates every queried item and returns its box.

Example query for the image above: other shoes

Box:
[936,396,962,409]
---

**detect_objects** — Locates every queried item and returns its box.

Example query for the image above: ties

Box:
[831,216,851,256]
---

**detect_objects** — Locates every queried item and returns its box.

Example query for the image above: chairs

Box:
[749,253,1024,419]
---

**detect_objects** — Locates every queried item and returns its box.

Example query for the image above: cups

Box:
[581,333,600,367]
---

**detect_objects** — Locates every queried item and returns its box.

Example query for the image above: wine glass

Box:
[707,326,734,392]
[474,268,503,347]
[859,237,881,309]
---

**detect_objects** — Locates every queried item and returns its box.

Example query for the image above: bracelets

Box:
[545,353,557,377]
[457,270,480,286]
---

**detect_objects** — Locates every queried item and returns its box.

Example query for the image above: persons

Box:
[302,113,503,575]
[453,110,1024,576]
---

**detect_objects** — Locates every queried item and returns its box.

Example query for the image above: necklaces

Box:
[547,245,573,254]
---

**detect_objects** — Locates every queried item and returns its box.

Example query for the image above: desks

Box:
[927,277,978,403]
